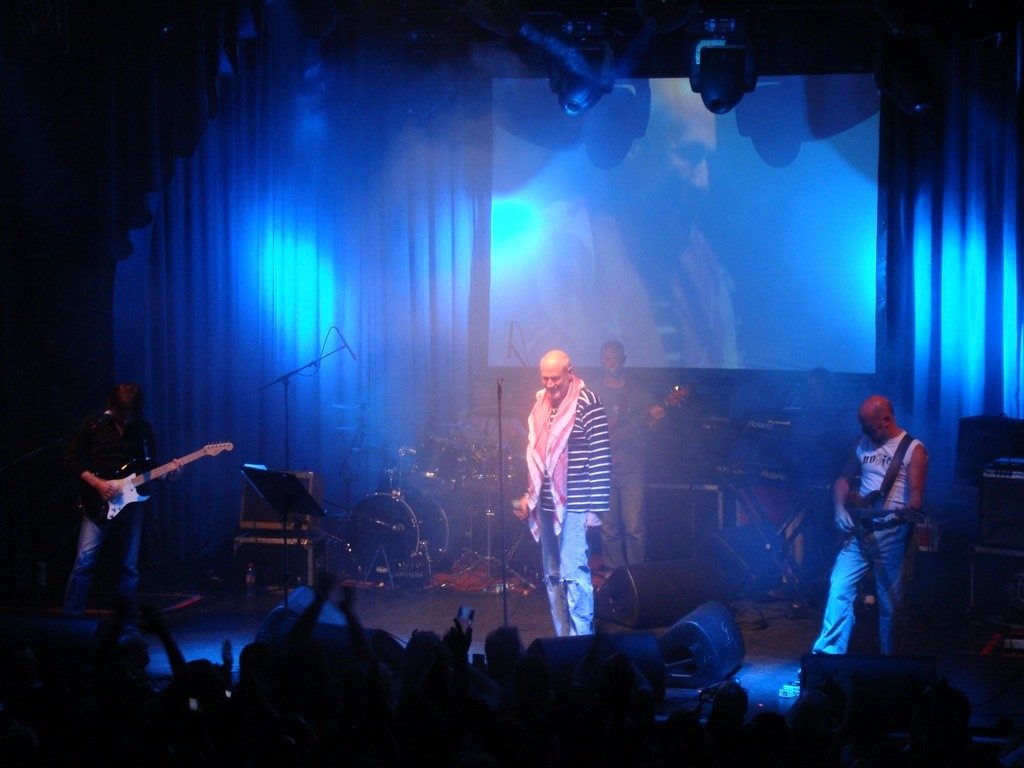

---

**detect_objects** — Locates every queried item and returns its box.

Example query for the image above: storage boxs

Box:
[641,482,736,556]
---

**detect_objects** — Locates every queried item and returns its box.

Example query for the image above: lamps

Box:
[545,31,625,114]
[687,20,768,117]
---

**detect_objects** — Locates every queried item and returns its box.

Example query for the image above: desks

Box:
[232,531,324,590]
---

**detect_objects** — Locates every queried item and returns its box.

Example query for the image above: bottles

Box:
[483,581,516,594]
[244,563,257,599]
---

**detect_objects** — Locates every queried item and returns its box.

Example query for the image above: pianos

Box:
[680,410,856,618]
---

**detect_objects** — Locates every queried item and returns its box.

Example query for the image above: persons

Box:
[597,342,662,571]
[544,77,735,368]
[0,572,1024,767]
[59,365,183,617]
[512,350,611,637]
[779,396,928,696]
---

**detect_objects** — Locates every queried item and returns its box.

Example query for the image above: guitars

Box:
[79,441,234,526]
[611,387,691,451]
[836,489,926,543]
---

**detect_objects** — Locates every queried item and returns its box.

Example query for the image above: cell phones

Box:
[457,605,475,633]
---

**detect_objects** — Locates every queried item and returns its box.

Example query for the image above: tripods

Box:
[434,482,540,593]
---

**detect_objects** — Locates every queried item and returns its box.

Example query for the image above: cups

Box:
[865,595,875,606]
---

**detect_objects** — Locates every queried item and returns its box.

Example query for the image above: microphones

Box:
[511,499,523,513]
[508,323,513,359]
[334,327,357,360]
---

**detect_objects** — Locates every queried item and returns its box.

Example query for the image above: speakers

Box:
[525,628,668,682]
[800,653,940,731]
[239,471,322,535]
[594,562,719,628]
[0,618,101,686]
[983,457,1024,549]
[269,620,407,676]
[657,599,747,689]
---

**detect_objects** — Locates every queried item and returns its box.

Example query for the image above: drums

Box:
[471,449,513,482]
[407,438,468,489]
[344,483,450,578]
